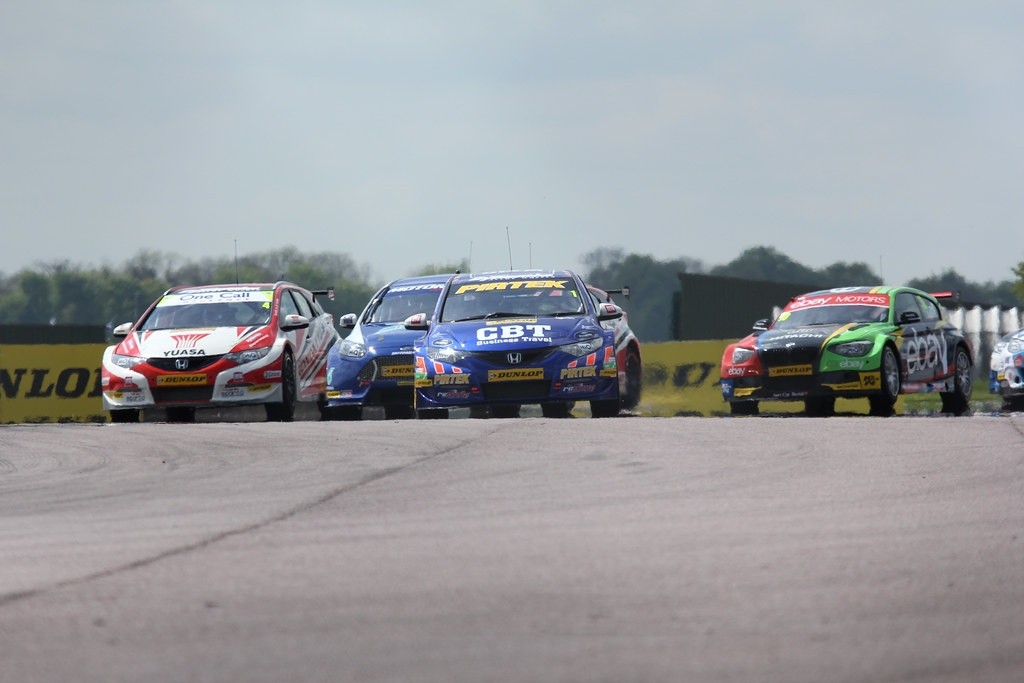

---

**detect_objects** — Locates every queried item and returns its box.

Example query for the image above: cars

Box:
[326,274,449,419]
[405,268,623,419]
[720,285,974,415]
[102,280,343,423]
[989,328,1024,407]
[493,285,642,418]
